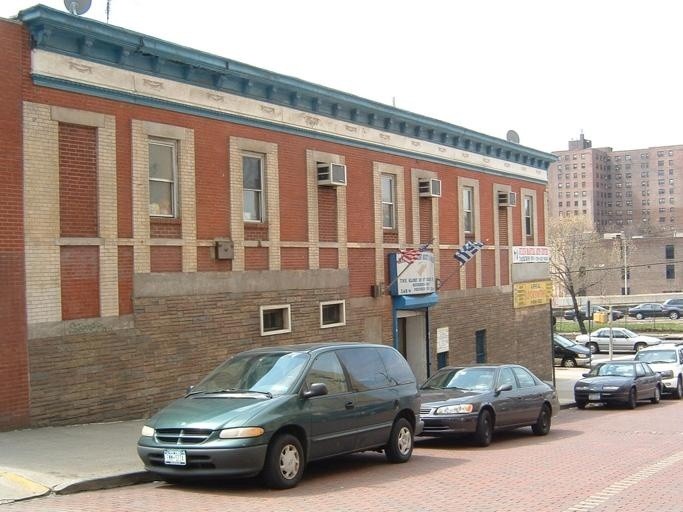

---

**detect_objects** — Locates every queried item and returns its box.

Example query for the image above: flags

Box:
[452,241,482,263]
[397,246,425,263]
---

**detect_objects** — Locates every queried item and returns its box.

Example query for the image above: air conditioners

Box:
[419,178,442,198]
[318,163,347,186]
[498,191,516,207]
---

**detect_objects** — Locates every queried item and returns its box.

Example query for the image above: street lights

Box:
[603,231,629,297]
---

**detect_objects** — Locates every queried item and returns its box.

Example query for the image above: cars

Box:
[572,361,665,411]
[628,302,668,319]
[414,362,559,449]
[562,304,624,323]
[574,326,661,354]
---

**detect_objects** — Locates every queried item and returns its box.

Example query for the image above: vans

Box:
[137,339,423,491]
[552,331,593,368]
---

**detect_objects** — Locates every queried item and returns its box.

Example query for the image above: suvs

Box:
[631,342,682,400]
[660,298,682,320]
